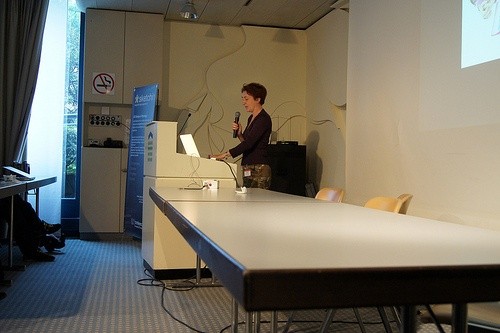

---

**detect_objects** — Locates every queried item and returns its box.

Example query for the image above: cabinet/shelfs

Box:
[79,6,166,238]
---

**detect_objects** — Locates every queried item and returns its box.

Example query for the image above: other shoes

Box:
[42,220,61,234]
[32,247,55,262]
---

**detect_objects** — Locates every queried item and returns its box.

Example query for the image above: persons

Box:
[0,194,65,262]
[207,82,272,189]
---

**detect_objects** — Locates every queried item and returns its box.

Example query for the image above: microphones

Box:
[233,112,240,138]
[177,113,191,136]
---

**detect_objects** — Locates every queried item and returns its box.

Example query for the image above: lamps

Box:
[178,1,199,20]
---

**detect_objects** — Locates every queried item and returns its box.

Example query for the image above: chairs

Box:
[283,180,413,333]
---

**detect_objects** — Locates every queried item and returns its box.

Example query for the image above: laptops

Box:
[180,134,200,157]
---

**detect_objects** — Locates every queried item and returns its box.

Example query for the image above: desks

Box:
[0,174,59,288]
[148,182,332,333]
[163,200,500,333]
